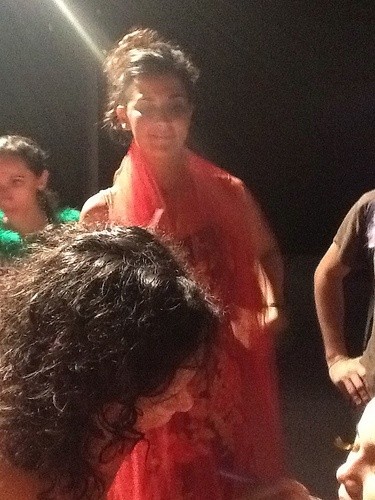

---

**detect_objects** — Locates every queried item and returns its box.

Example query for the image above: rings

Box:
[347,387,351,392]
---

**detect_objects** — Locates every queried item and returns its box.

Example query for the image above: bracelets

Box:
[328,354,346,370]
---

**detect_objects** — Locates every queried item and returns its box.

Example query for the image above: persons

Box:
[0,135,80,259]
[79,27,287,500]
[315,188,375,423]
[336,397,375,500]
[0,227,222,500]
